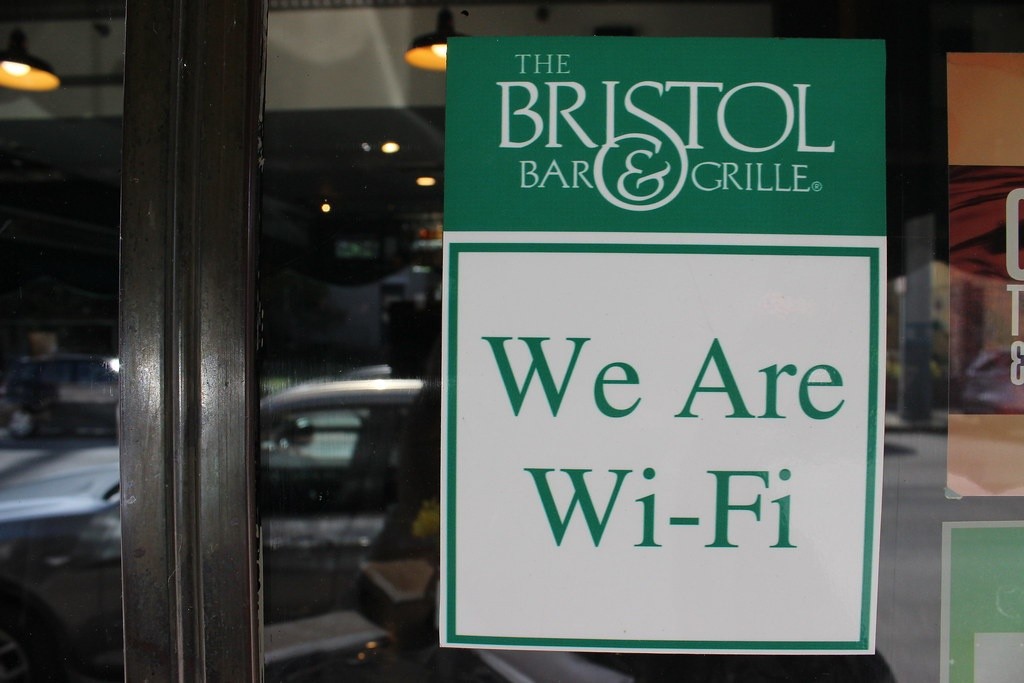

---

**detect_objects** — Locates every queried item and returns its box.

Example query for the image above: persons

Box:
[387,282,418,378]
[354,383,441,605]
[949,281,1024,413]
[337,418,399,472]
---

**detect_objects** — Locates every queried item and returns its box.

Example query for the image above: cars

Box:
[0,380,442,683]
[963,349,1024,415]
[0,354,123,440]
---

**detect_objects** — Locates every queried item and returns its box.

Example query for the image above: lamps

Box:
[0,27,62,93]
[402,8,471,73]
